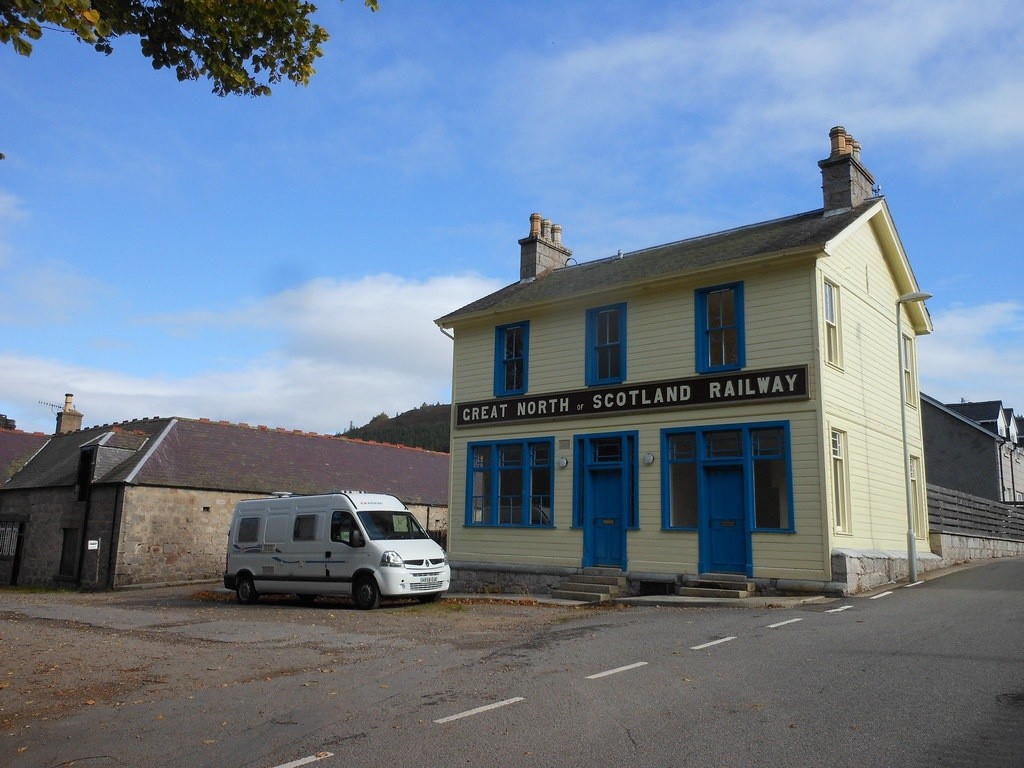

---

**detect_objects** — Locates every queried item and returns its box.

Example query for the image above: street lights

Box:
[895,293,932,584]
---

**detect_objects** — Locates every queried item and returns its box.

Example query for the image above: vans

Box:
[223,490,451,610]
